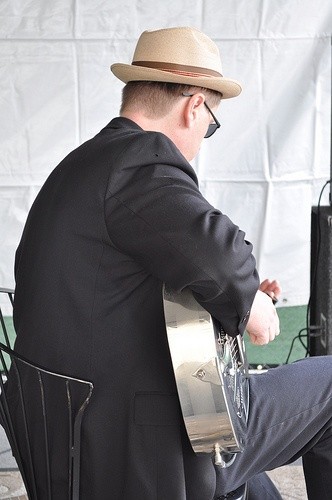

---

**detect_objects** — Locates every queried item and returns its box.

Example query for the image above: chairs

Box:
[0,342,249,500]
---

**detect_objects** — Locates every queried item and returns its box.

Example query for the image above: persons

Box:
[4,25,331,500]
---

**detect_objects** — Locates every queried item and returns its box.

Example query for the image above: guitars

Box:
[163,280,249,456]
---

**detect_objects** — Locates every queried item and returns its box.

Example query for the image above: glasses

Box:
[182,93,220,139]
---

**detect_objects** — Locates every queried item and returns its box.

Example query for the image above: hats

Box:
[111,26,242,100]
